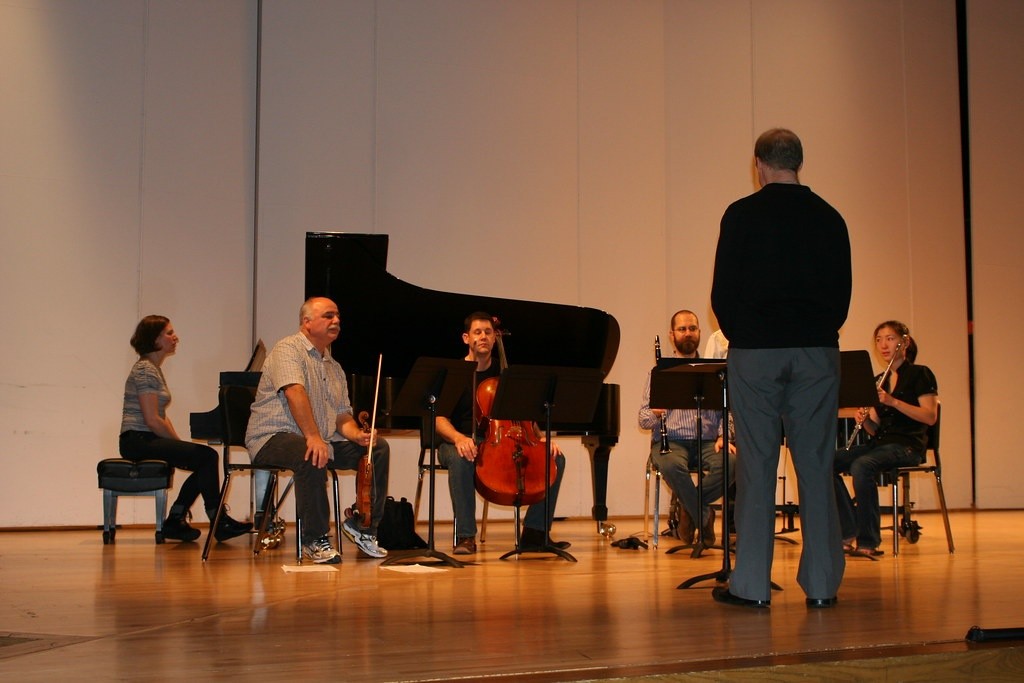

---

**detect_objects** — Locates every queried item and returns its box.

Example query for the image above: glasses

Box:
[673,325,699,334]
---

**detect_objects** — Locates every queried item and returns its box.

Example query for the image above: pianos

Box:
[188,231,623,539]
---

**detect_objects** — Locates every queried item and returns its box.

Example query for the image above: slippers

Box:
[856,545,885,556]
[843,539,857,554]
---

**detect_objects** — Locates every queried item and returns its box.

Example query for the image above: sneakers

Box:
[522,529,571,550]
[303,534,342,564]
[164,516,201,540]
[211,515,253,541]
[341,518,388,557]
[699,508,717,547]
[677,509,696,544]
[454,538,477,555]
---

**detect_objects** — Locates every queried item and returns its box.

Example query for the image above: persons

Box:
[711,128,852,609]
[245,297,390,564]
[432,311,571,554]
[832,320,939,556]
[639,309,737,550]
[704,329,737,544]
[118,314,254,541]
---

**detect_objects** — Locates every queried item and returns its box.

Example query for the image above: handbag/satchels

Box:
[378,496,415,550]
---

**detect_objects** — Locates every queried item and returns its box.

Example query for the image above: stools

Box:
[97,458,175,545]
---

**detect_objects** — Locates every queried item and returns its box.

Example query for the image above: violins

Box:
[353,409,382,531]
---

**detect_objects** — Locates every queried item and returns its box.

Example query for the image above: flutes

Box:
[654,334,672,456]
[844,334,907,453]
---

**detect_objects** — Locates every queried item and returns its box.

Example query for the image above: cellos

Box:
[473,315,559,561]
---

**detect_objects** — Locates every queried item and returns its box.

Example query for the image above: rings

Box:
[462,447,465,450]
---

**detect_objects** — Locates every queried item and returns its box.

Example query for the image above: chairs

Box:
[414,415,520,549]
[643,429,722,547]
[201,384,342,560]
[893,399,955,554]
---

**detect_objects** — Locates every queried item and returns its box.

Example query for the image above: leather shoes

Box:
[712,585,770,609]
[806,597,837,608]
